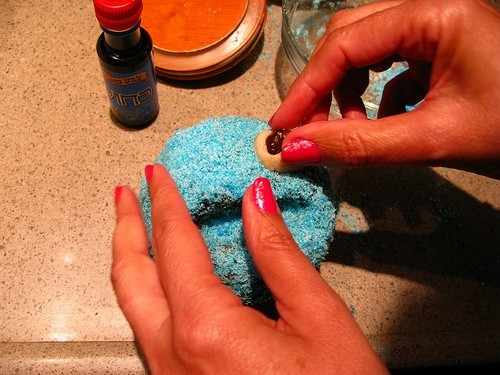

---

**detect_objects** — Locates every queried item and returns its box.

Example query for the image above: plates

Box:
[140,0,267,81]
[281,0,426,122]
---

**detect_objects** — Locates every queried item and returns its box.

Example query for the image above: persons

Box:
[111,0,500,375]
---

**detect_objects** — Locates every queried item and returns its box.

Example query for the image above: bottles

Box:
[93,0,159,129]
